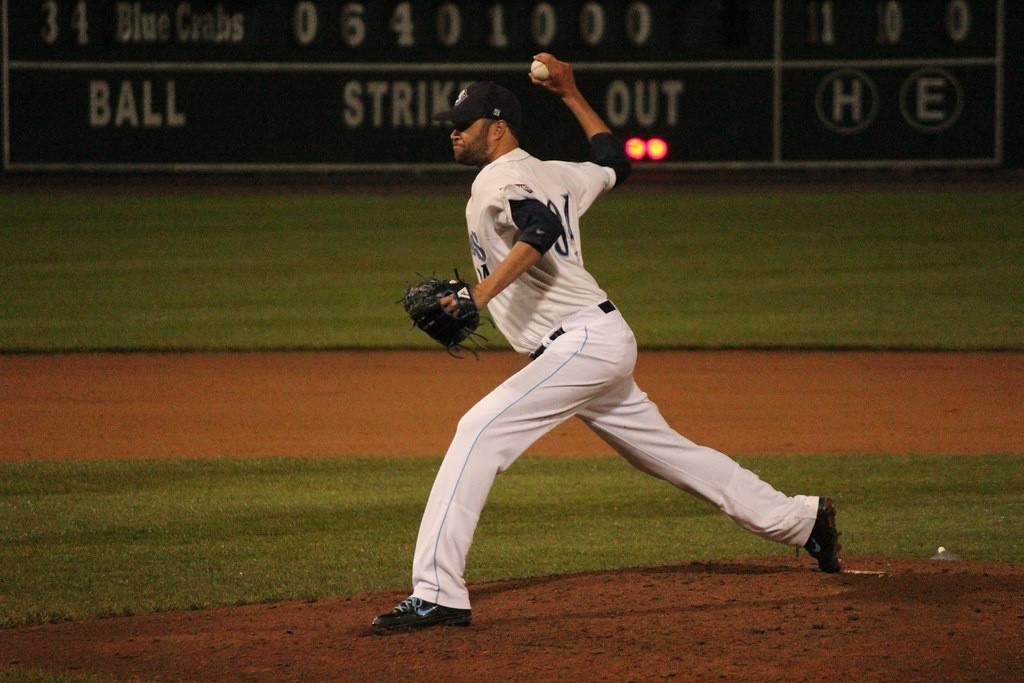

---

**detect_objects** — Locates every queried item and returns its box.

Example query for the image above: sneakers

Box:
[372,597,472,634]
[804,496,840,573]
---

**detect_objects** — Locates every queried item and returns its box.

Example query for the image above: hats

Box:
[429,81,522,134]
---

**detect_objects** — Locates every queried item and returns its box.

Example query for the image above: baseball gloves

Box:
[400,275,481,351]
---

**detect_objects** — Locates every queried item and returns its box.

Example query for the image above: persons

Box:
[370,53,842,635]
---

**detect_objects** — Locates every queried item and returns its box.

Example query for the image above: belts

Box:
[529,301,615,360]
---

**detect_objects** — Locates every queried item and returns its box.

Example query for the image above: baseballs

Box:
[528,55,556,85]
